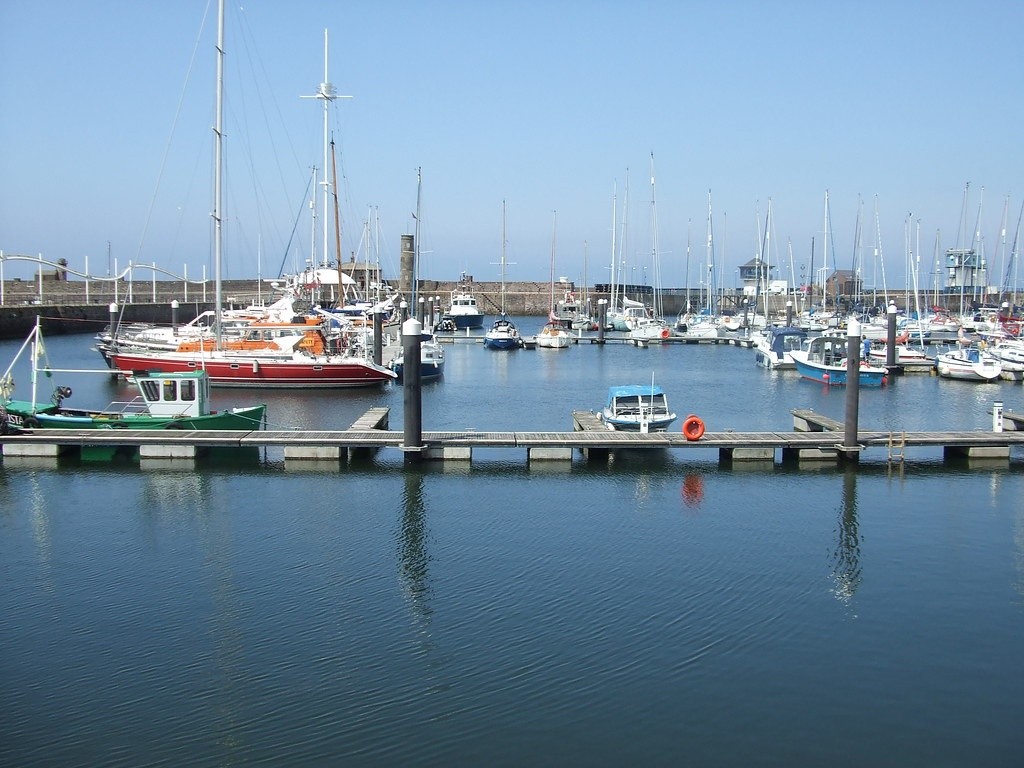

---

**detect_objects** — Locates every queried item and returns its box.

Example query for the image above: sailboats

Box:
[482,200,525,350]
[93,0,449,393]
[537,177,1023,390]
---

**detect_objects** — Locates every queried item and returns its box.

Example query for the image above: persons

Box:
[862,334,872,359]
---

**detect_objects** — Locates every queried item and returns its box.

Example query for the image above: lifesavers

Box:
[337,339,343,352]
[662,330,670,338]
[683,418,705,440]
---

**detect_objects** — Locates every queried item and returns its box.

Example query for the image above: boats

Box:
[602,372,678,433]
[0,315,267,465]
[441,270,486,330]
